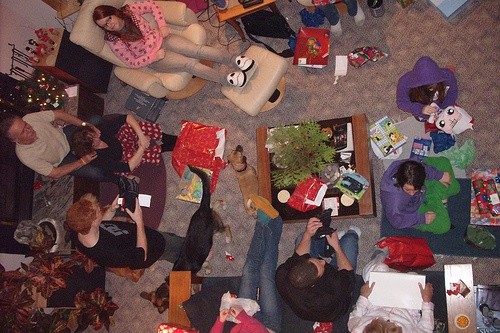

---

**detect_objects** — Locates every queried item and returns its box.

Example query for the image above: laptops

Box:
[367,271,426,310]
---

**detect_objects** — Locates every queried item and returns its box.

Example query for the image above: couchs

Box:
[69,0,213,99]
[169,263,477,333]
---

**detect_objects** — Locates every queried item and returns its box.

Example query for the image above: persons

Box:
[381,157,460,233]
[347,236,435,332]
[91,0,254,88]
[66,194,188,268]
[322,1,365,36]
[208,194,282,333]
[395,56,459,122]
[274,219,360,320]
[70,115,177,170]
[4,111,142,182]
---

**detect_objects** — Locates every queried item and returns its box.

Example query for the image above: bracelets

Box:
[80,158,87,165]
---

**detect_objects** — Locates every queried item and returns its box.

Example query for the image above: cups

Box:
[277,190,290,203]
[340,194,355,206]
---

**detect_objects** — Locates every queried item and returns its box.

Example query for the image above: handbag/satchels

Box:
[241,10,297,58]
[374,235,436,271]
[287,177,327,212]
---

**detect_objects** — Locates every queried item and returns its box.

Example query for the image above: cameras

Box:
[315,208,334,240]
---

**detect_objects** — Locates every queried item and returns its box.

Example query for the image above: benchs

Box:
[34,83,104,254]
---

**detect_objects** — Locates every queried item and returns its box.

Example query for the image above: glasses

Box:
[103,15,112,30]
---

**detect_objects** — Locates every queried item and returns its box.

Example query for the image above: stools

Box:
[221,45,289,117]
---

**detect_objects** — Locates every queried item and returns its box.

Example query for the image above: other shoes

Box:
[354,1,365,26]
[249,193,279,220]
[235,55,254,72]
[329,21,343,36]
[226,71,246,87]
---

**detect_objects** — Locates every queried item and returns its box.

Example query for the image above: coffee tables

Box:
[257,113,377,223]
[213,0,279,43]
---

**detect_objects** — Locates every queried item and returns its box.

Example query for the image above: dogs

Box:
[227,144,259,220]
[139,163,233,315]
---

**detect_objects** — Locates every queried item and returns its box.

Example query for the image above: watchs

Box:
[82,122,86,127]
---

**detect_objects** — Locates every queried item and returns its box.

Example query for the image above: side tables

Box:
[32,28,112,94]
[36,264,105,309]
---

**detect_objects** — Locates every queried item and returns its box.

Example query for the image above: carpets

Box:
[380,178,500,258]
[74,114,167,230]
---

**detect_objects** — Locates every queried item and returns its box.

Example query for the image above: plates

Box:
[455,314,470,329]
